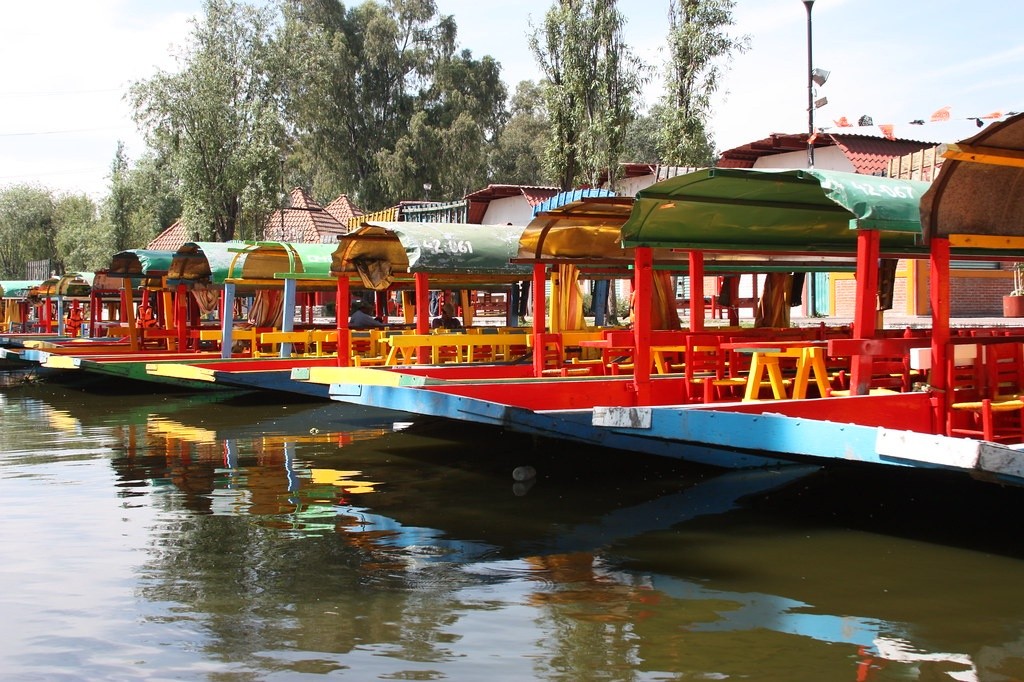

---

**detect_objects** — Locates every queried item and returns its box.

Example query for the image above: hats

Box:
[360,302,372,309]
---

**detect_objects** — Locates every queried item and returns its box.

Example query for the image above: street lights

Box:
[800,0,820,317]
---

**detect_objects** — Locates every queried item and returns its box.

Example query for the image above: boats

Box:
[0,165,931,473]
[591,106,1024,496]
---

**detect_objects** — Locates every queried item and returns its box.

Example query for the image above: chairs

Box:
[0,306,1024,444]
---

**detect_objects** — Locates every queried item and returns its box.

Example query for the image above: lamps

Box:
[812,67,831,87]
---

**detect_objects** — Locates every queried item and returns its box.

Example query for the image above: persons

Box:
[431,303,461,329]
[350,301,395,327]
